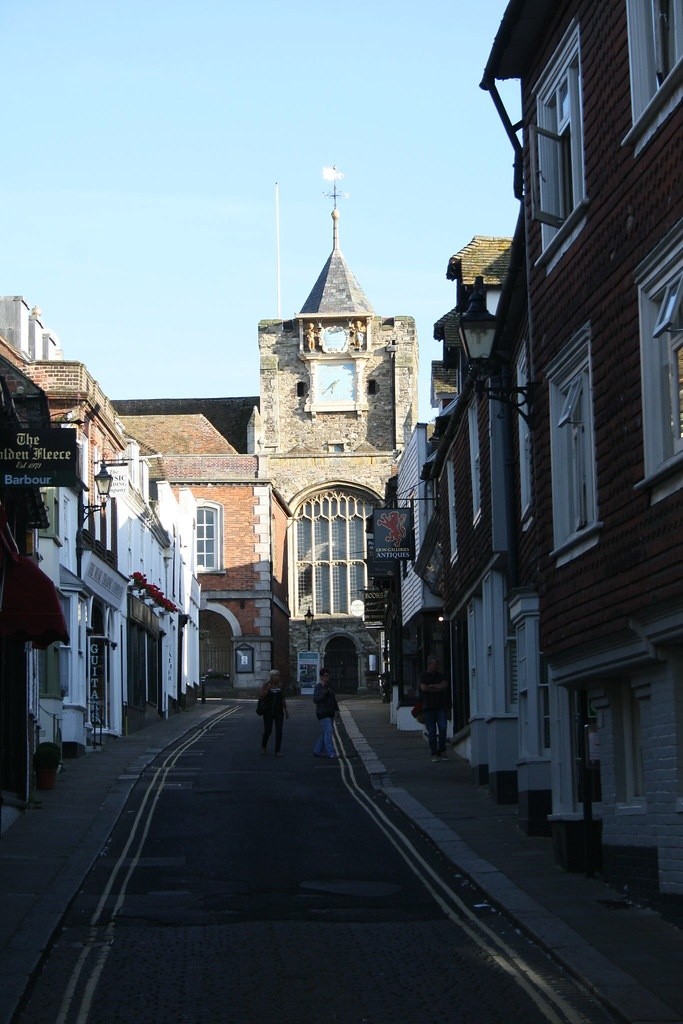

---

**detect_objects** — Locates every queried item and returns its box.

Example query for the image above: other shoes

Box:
[328,755,337,760]
[277,752,285,757]
[262,751,272,755]
[441,753,449,761]
[313,751,322,756]
[432,755,438,762]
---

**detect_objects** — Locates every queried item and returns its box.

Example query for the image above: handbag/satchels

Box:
[256,695,264,715]
[412,696,426,725]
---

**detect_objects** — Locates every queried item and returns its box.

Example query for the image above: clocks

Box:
[316,363,356,401]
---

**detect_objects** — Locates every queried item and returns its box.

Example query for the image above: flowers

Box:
[132,572,178,613]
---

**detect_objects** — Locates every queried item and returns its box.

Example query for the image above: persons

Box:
[255,668,291,757]
[314,668,342,759]
[418,655,451,763]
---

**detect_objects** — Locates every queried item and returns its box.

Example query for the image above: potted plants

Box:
[33,742,61,789]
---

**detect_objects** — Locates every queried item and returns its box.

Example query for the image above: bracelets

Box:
[285,708,288,711]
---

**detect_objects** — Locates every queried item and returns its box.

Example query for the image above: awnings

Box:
[0,549,72,650]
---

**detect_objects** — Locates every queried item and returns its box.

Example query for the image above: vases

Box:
[128,579,140,590]
[149,598,159,608]
[158,606,168,614]
[139,589,151,599]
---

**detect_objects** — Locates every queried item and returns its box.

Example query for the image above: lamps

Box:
[457,276,537,432]
[83,458,113,524]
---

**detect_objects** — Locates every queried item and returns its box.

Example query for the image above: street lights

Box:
[304,606,314,652]
